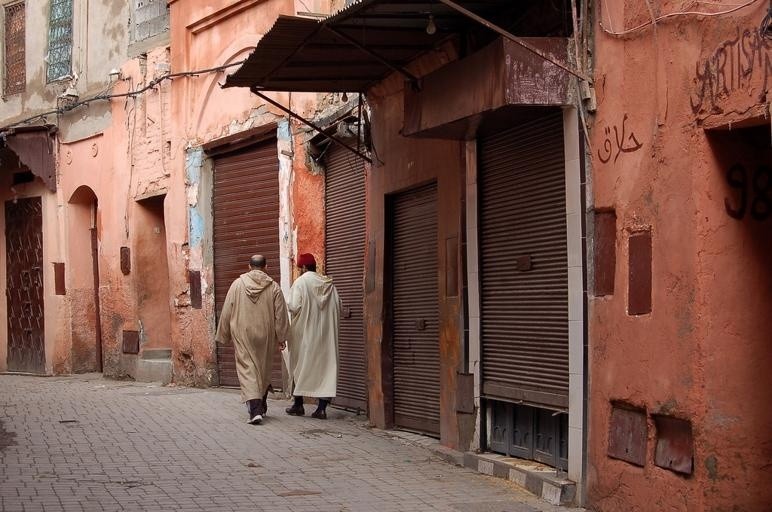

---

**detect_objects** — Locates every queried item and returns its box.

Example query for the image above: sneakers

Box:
[247,413,266,423]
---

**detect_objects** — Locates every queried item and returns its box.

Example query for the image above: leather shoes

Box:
[286,405,305,415]
[312,409,326,418]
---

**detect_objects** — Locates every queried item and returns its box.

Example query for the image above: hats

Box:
[297,253,316,266]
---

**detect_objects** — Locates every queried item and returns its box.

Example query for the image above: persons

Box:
[214,254,290,424]
[285,252,345,419]
